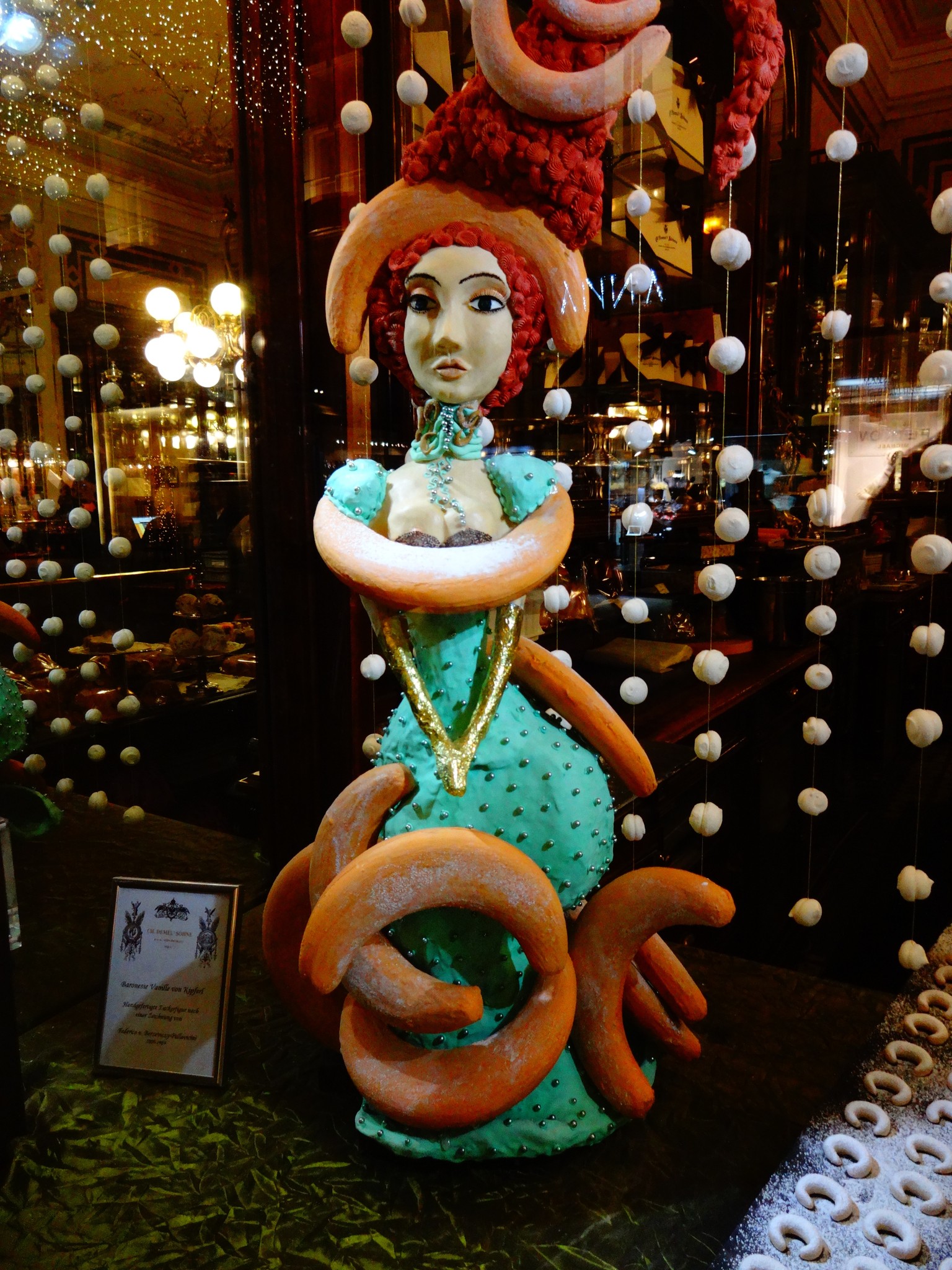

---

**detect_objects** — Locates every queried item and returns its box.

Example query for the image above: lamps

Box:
[143,195,246,388]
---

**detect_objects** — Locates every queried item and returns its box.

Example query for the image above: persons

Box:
[259,0,785,1167]
